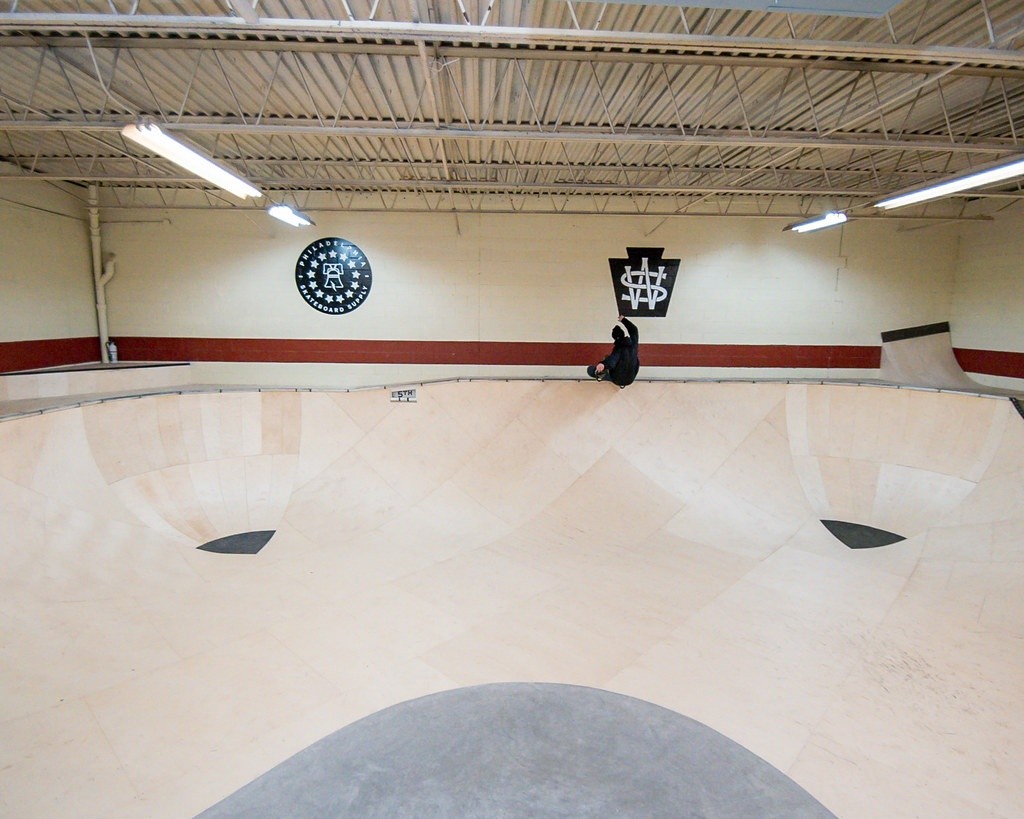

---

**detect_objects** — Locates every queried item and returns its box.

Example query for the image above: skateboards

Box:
[587,364,609,381]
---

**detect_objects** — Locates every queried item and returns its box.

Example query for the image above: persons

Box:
[587,315,640,388]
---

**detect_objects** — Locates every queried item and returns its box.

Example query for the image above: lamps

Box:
[266,204,311,227]
[863,158,1024,211]
[122,122,265,199]
[781,212,848,234]
